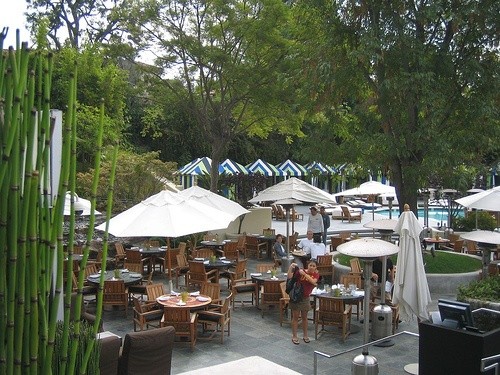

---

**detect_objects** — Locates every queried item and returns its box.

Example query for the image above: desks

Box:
[192,257,234,292]
[200,240,227,257]
[256,234,284,256]
[136,247,167,271]
[311,289,365,334]
[251,271,290,285]
[157,294,212,341]
[87,270,142,311]
[424,238,450,250]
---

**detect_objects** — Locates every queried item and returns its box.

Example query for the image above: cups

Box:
[324,284,329,292]
[333,283,356,296]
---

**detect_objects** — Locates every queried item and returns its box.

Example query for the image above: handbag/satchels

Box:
[288,281,304,304]
[285,266,299,294]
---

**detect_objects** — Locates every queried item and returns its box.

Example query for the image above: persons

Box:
[273,233,288,259]
[297,231,314,259]
[373,255,394,297]
[311,235,326,260]
[307,207,325,237]
[287,259,319,344]
[320,206,330,247]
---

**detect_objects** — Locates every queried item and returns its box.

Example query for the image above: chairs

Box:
[66,229,483,375]
[271,199,382,224]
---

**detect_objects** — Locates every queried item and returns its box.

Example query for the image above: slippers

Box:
[303,337,310,343]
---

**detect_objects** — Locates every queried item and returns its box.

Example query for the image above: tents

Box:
[173,157,362,200]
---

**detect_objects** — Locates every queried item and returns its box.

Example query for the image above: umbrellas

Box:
[454,186,500,211]
[177,182,251,223]
[95,185,235,292]
[248,177,336,257]
[332,181,396,239]
[392,204,432,330]
